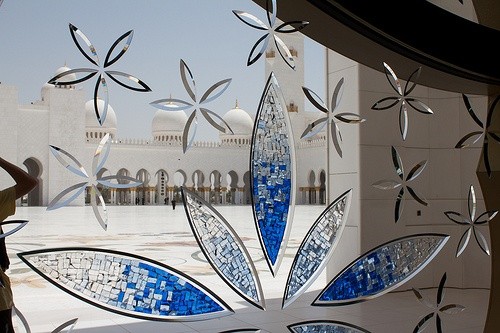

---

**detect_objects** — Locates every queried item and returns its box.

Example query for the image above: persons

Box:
[130,192,176,210]
[0,155,38,333]
